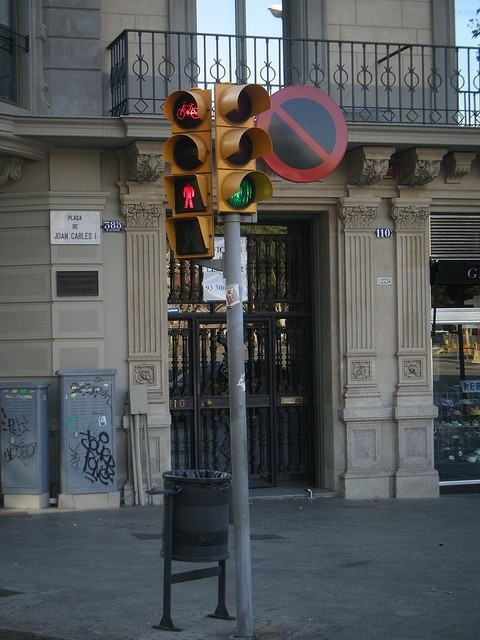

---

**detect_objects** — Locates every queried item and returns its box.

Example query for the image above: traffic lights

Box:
[214,82,274,214]
[162,87,215,260]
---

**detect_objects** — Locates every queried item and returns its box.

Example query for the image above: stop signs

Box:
[256,84,348,183]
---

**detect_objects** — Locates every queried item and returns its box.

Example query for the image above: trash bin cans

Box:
[160,469,232,562]
